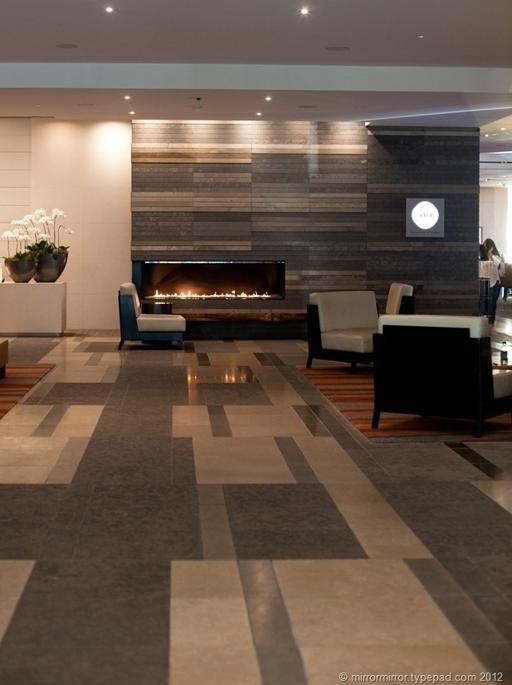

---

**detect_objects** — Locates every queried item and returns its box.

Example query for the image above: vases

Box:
[24,249,69,284]
[3,253,40,285]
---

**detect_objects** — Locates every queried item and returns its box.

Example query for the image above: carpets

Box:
[0,361,56,423]
[290,359,512,445]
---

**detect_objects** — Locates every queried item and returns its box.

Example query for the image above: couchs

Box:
[478,261,512,321]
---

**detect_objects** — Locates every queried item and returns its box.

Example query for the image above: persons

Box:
[484,239,501,324]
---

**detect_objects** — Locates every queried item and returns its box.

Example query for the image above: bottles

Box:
[500,342,508,366]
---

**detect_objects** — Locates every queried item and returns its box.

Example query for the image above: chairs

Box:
[367,309,512,438]
[382,281,417,315]
[111,282,187,351]
[301,290,380,371]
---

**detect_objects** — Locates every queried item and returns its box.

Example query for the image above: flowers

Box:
[0,204,78,257]
